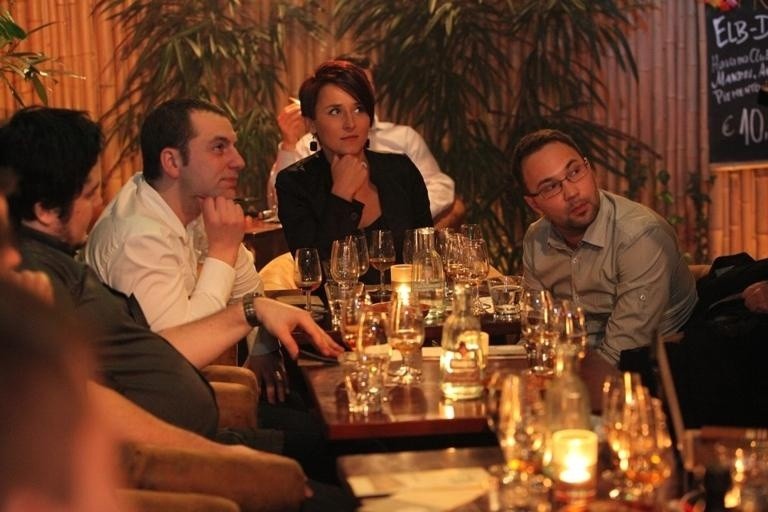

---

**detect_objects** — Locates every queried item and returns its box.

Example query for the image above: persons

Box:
[511,129,699,369]
[741,280,768,314]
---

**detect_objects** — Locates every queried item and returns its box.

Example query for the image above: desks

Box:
[264,284,680,511]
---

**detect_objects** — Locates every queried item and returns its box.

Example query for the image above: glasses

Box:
[527,159,588,200]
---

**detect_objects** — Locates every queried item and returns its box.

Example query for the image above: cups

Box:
[293,223,768,512]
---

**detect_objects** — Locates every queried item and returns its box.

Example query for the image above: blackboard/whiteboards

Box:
[705,0,768,173]
